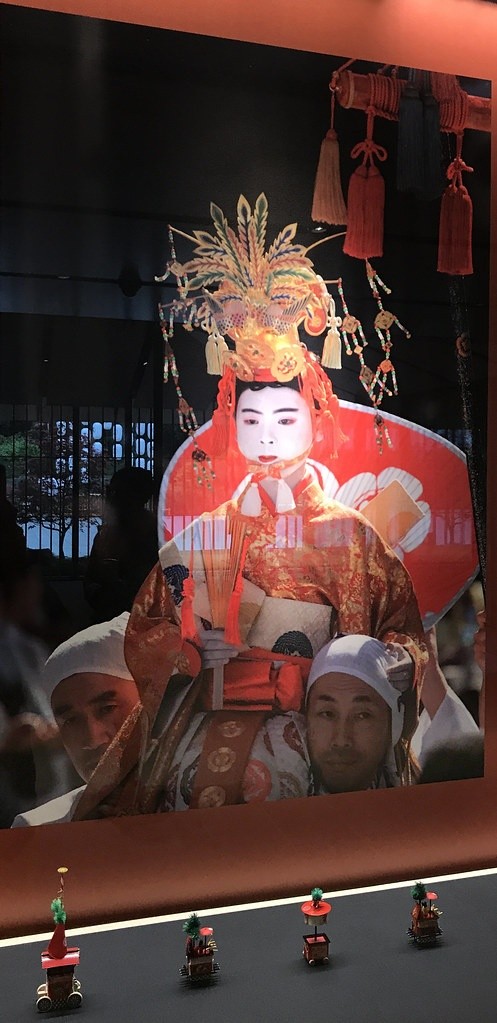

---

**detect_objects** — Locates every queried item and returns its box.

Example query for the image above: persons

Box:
[1,465,485,832]
[71,379,421,824]
